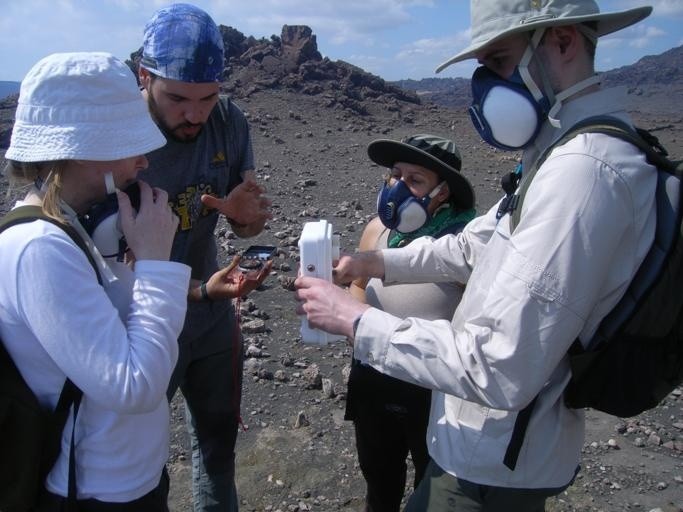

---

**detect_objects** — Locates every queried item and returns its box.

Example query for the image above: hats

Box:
[364,132,477,214]
[138,2,226,85]
[4,49,170,162]
[430,0,654,77]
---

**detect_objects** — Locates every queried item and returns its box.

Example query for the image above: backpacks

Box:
[496,111,683,420]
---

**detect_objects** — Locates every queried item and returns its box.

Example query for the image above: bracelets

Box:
[199,278,209,302]
[225,214,247,228]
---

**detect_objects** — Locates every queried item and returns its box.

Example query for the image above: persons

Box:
[338,131,476,511]
[81,2,274,512]
[293,0,659,512]
[0,49,272,510]
